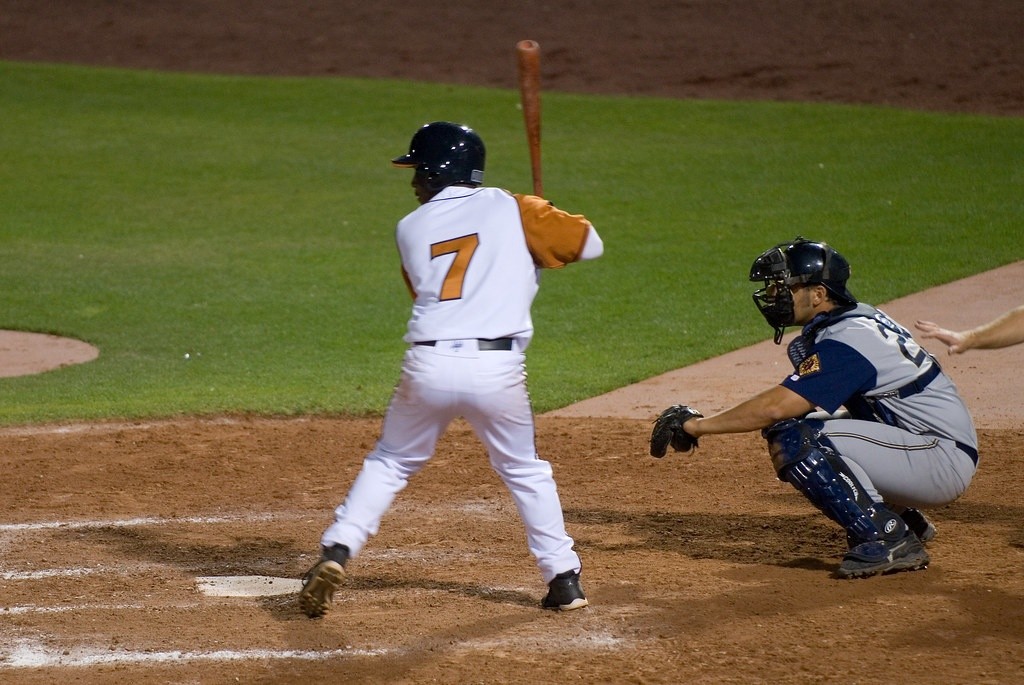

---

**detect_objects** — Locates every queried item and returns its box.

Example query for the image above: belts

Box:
[416,338,518,352]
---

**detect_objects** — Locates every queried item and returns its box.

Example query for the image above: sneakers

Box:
[538,571,588,610]
[300,544,348,618]
[837,508,937,580]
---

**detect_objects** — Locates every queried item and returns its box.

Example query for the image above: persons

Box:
[298,122,606,619]
[914,304,1024,355]
[650,240,980,576]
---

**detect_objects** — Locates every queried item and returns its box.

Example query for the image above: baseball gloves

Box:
[646,404,705,460]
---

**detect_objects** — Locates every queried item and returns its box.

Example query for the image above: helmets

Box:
[750,234,858,306]
[389,121,489,189]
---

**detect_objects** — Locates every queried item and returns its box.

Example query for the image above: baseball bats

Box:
[513,36,546,198]
